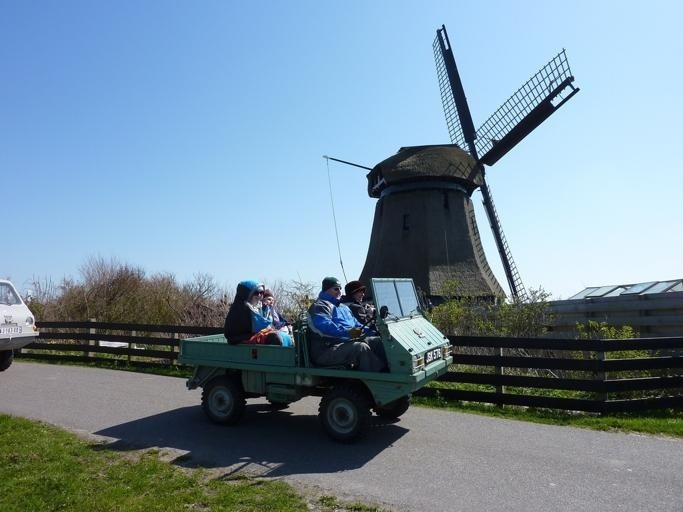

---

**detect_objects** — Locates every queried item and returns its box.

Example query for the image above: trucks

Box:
[177,274,454,443]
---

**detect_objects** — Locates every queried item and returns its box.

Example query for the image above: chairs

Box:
[302,334,352,370]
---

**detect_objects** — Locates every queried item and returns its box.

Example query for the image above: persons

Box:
[339,280,379,337]
[305,276,390,374]
[223,281,296,348]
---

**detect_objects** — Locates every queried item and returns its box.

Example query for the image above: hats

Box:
[345,282,366,295]
[323,276,340,291]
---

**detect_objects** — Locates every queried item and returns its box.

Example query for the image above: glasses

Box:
[333,287,342,291]
[254,291,264,296]
